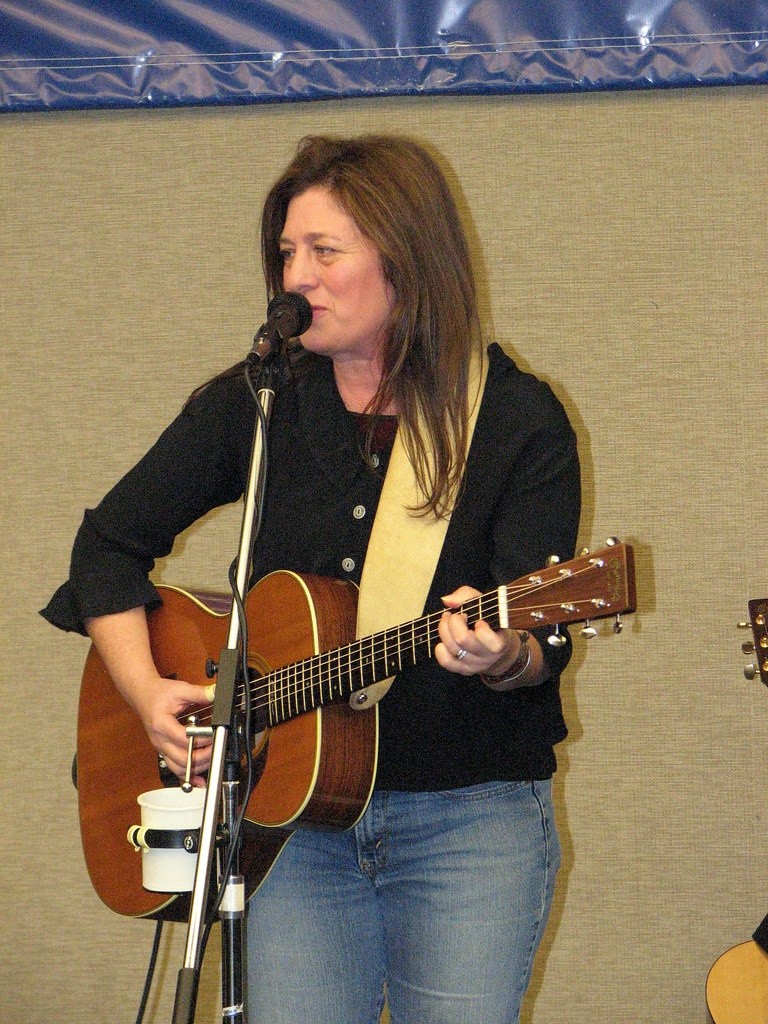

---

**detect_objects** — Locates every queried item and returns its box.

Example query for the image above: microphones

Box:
[244,291,313,368]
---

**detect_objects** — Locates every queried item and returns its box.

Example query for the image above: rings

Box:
[455,650,466,660]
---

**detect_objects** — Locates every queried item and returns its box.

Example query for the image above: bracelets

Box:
[480,632,532,687]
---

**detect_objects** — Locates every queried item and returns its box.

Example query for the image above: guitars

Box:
[705,598,768,1024]
[75,535,638,919]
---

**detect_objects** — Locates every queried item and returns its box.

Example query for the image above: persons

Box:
[40,134,581,1024]
